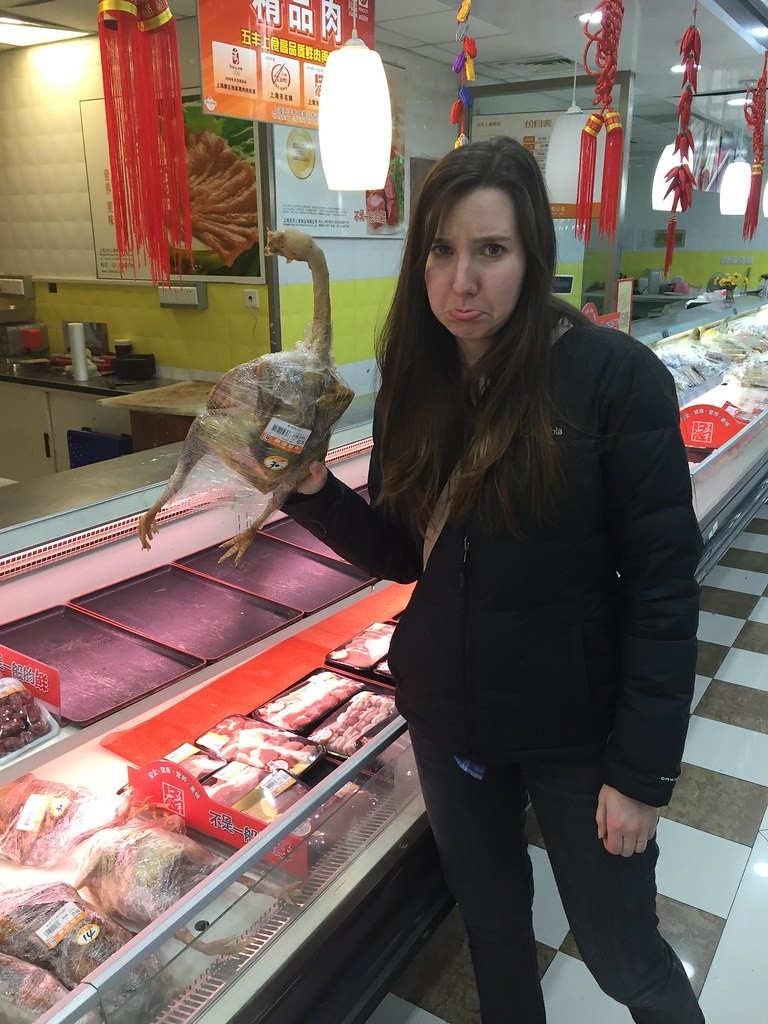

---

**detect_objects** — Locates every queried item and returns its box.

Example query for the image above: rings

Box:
[636,841,647,845]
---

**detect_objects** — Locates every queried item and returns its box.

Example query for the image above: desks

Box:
[96,379,218,453]
[582,292,697,320]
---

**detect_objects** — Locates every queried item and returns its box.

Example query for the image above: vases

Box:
[727,291,735,302]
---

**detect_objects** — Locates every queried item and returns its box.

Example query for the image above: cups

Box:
[115,339,131,356]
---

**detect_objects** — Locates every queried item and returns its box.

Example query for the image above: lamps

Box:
[545,11,606,204]
[719,79,758,216]
[652,115,693,212]
[318,0,392,191]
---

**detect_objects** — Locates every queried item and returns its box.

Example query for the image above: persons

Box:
[280,137,708,1024]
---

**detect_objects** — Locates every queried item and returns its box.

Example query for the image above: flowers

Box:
[713,272,749,291]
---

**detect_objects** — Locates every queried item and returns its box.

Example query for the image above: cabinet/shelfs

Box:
[0,380,132,481]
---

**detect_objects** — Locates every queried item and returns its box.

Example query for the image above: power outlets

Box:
[158,286,198,305]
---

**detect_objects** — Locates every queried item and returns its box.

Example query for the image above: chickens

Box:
[136,228,355,565]
